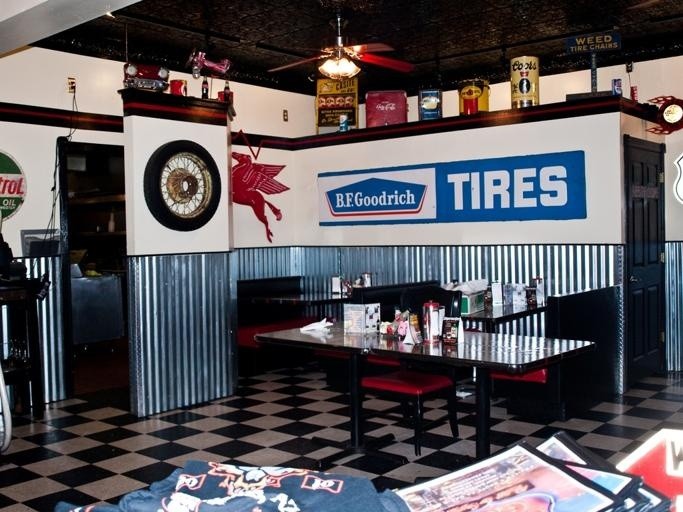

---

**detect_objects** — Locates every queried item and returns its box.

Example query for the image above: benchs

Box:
[488,287,616,421]
[236,275,472,381]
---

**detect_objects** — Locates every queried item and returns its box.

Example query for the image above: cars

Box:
[339,115,348,133]
[417,90,442,122]
[630,86,638,102]
[169,80,186,97]
[457,78,491,114]
[510,57,540,109]
[612,78,620,96]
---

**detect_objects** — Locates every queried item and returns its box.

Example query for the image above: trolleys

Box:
[267,15,415,78]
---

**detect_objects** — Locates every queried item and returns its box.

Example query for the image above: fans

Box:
[267,15,415,78]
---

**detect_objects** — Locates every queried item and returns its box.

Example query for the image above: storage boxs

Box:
[364,89,409,131]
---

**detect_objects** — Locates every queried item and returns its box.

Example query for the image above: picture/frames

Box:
[0,275,49,428]
[71,273,122,355]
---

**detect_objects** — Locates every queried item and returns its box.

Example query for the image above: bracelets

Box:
[122,79,168,92]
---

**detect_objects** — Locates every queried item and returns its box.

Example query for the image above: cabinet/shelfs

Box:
[486,277,544,308]
[223,79,229,100]
[378,298,446,344]
[65,192,126,239]
[340,270,372,297]
[199,75,208,98]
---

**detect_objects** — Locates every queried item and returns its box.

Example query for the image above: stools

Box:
[352,363,460,456]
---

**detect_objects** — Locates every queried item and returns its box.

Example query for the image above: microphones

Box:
[352,363,460,456]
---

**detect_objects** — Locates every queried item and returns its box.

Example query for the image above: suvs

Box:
[457,78,491,117]
[509,55,540,110]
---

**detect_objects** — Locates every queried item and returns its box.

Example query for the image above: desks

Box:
[239,317,598,464]
[0,275,49,428]
[71,273,122,355]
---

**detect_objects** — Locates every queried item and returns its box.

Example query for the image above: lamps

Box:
[315,52,361,78]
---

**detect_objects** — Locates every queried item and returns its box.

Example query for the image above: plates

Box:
[65,192,126,239]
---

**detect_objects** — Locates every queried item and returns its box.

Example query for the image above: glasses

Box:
[315,52,361,78]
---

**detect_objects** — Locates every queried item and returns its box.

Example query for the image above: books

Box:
[364,89,409,131]
